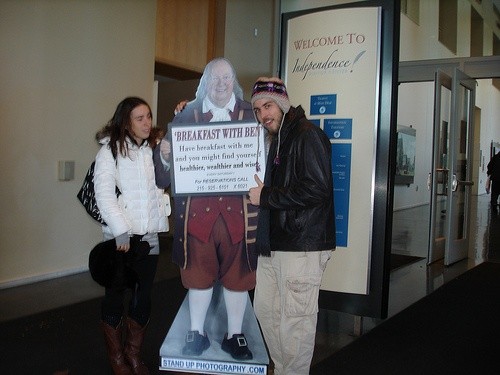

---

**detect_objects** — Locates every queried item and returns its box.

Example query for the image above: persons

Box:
[484,152,500,224]
[175,76,337,375]
[96,97,172,375]
[160,57,271,361]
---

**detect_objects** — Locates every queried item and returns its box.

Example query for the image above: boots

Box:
[100,315,132,375]
[124,312,152,374]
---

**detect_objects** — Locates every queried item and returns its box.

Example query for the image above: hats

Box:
[88,238,150,290]
[250,77,291,173]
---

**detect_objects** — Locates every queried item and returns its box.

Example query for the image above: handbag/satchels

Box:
[76,140,122,227]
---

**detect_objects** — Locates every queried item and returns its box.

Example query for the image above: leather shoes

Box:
[182,330,211,357]
[221,332,254,360]
